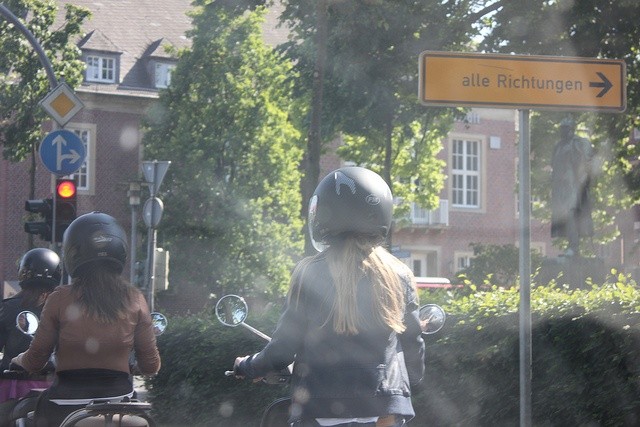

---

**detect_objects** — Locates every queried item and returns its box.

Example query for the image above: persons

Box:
[12,211,161,426]
[232,165,426,426]
[0,248,62,398]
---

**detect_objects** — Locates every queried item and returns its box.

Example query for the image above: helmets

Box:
[16,248,61,289]
[63,211,128,278]
[308,167,393,253]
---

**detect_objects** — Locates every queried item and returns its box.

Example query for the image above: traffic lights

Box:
[56,179,78,242]
[24,197,52,243]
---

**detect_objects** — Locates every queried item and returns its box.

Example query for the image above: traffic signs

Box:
[418,52,626,115]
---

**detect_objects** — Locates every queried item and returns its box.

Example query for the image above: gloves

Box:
[11,353,31,376]
[233,354,254,384]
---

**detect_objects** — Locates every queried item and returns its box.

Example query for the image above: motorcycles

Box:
[0,387,51,427]
[215,294,446,427]
[7,310,168,426]
